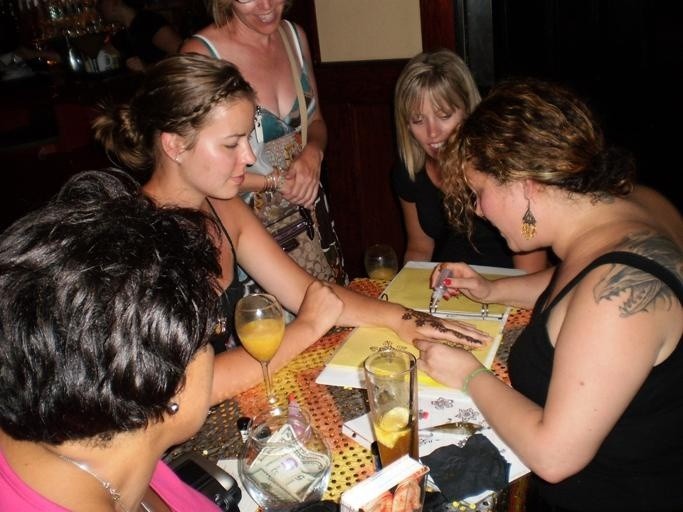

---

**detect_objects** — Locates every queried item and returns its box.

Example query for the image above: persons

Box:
[412,79,683,512]
[0,168,231,512]
[97,0,183,77]
[179,0,335,324]
[393,48,547,274]
[88,51,493,409]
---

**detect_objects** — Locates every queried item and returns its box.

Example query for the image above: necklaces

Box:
[59,455,153,512]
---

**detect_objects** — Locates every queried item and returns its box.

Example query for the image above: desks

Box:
[162,279,533,512]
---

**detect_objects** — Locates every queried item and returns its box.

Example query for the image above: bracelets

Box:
[263,165,286,193]
[463,368,488,391]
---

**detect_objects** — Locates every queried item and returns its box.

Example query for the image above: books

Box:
[316,260,527,401]
[341,392,531,504]
[339,453,430,512]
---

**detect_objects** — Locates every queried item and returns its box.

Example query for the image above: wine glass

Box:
[235,293,310,444]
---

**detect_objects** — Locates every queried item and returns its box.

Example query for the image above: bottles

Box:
[236,405,336,511]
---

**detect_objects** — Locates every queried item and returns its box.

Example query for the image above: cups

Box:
[364,244,398,282]
[363,350,423,471]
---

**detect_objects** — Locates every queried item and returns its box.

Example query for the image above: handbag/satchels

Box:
[251,204,333,282]
